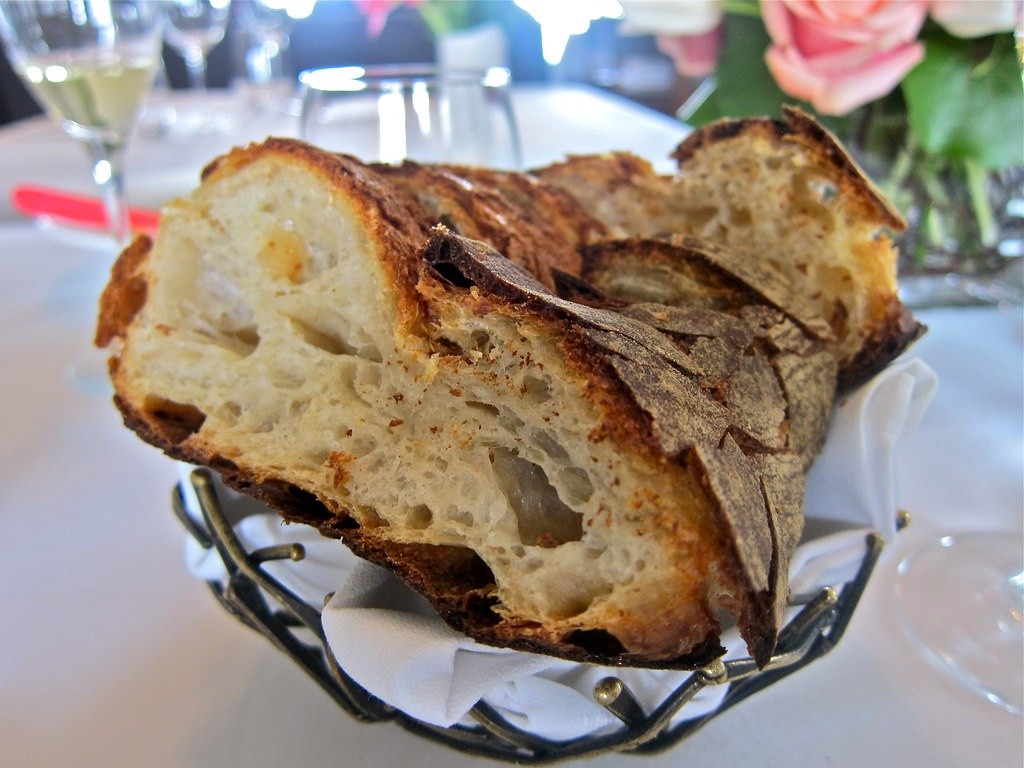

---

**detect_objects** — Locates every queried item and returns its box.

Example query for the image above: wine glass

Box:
[165,0,312,130]
[0,0,166,253]
[879,516,1024,768]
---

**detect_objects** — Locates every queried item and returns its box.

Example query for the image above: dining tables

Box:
[0,85,1024,768]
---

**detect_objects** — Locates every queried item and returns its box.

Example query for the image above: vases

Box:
[836,106,1024,314]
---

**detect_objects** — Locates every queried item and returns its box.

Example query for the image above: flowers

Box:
[676,0,1024,275]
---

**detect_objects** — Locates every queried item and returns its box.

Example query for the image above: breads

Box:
[94,103,923,666]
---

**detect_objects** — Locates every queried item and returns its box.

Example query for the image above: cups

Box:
[294,69,525,182]
